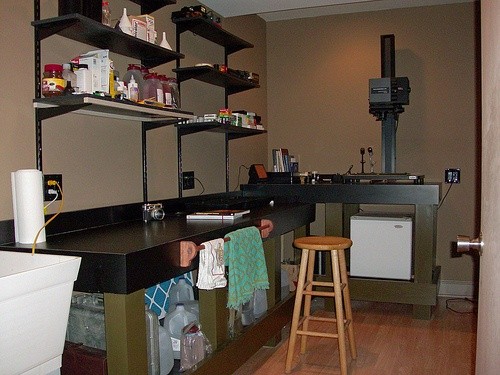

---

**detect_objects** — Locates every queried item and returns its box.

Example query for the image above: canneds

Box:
[41,64,65,98]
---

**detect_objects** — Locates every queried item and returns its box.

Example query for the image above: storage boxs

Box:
[281,263,300,291]
[70,49,115,98]
[204,112,264,131]
[247,72,260,83]
[128,14,155,44]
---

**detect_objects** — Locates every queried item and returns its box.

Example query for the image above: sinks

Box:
[0,250,83,375]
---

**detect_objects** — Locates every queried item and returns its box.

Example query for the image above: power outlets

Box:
[445,169,460,184]
[43,174,63,201]
[181,171,195,190]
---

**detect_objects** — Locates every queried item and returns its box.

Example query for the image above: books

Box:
[272,148,298,172]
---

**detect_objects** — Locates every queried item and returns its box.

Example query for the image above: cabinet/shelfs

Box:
[172,15,267,140]
[0,200,317,375]
[240,181,442,321]
[30,0,193,131]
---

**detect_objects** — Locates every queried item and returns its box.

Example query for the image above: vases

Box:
[159,31,172,50]
[119,8,132,35]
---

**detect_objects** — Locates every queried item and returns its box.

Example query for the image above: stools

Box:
[285,236,357,375]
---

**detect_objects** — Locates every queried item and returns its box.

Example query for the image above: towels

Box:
[195,237,228,290]
[224,226,270,310]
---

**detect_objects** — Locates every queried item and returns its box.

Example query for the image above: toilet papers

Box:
[10,168,46,245]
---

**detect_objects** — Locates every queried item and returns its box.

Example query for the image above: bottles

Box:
[281,268,289,300]
[42,62,77,98]
[311,170,319,182]
[145,278,204,375]
[154,30,158,45]
[115,63,177,109]
[119,7,132,34]
[102,1,112,27]
[160,31,172,50]
